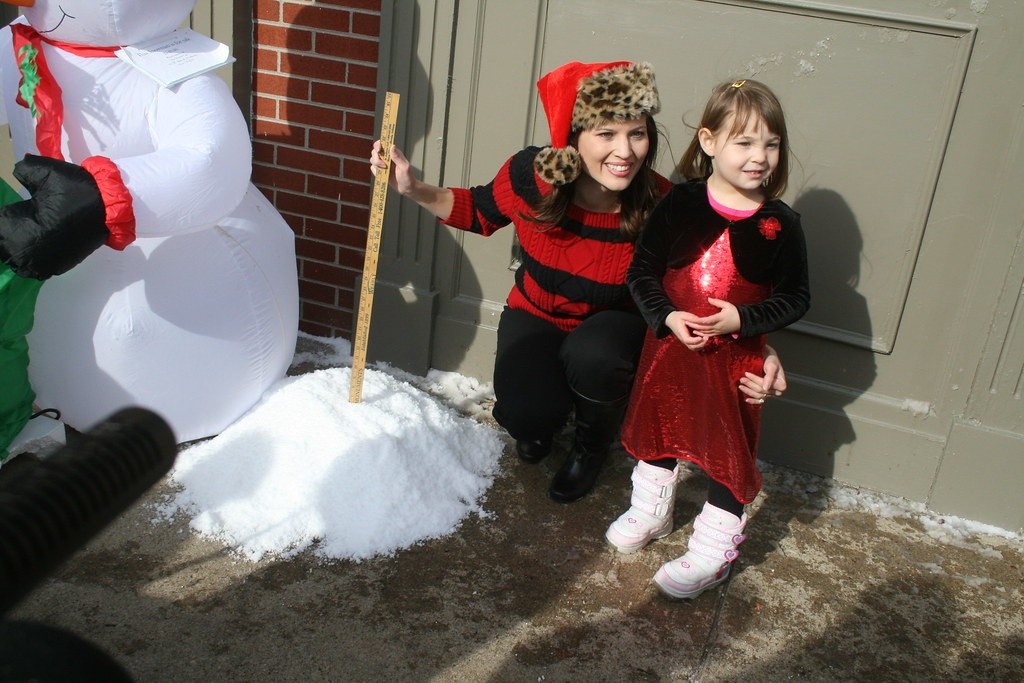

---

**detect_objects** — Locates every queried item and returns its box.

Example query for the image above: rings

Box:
[763,393,767,397]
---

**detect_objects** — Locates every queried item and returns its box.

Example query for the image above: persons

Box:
[603,61,812,603]
[369,61,786,503]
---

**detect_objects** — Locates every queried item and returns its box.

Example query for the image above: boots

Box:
[550,379,629,502]
[516,404,569,463]
[653,500,748,600]
[604,460,679,553]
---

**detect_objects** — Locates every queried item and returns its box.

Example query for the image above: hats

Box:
[533,62,661,185]
